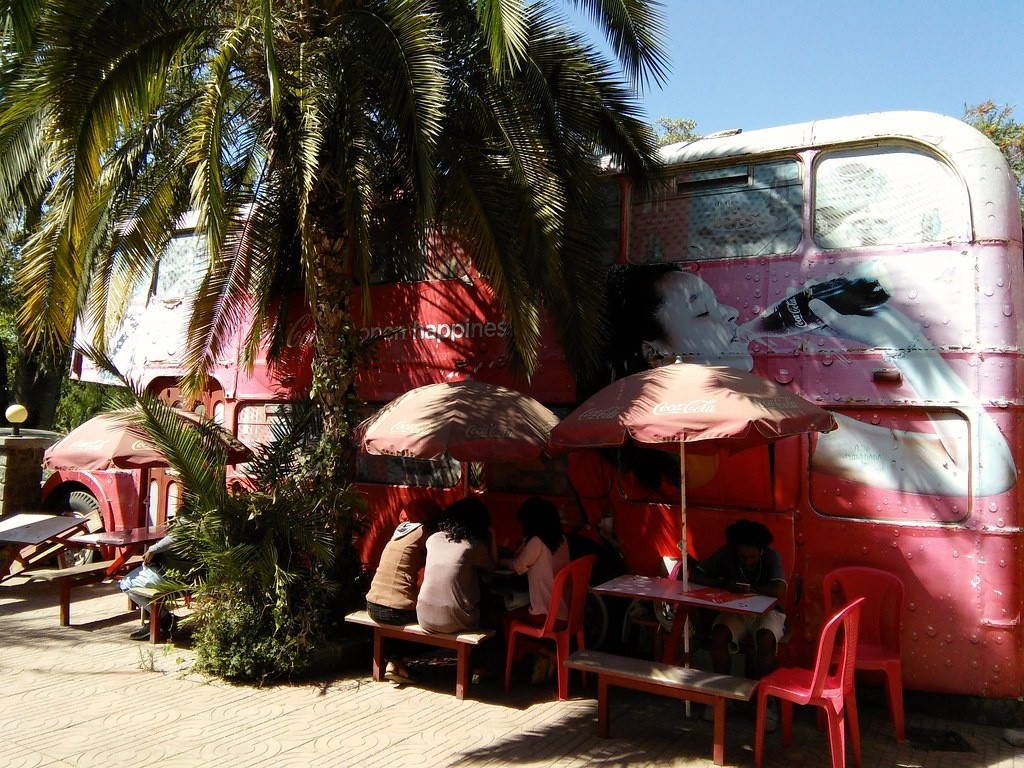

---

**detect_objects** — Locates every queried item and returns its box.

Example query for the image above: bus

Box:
[40,109,1022,725]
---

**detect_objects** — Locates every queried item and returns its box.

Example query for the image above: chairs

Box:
[817,566,905,744]
[755,598,865,768]
[504,555,596,702]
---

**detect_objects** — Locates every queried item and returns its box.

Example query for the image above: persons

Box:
[365,498,436,685]
[120,491,200,641]
[416,508,497,686]
[691,517,788,716]
[630,265,1017,499]
[496,499,577,684]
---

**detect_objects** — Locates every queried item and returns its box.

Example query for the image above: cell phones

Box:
[736,582,750,593]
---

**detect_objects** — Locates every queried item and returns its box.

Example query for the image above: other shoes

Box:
[130,614,175,639]
[763,703,778,733]
[532,650,557,683]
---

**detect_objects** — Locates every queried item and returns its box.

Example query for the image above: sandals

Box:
[383,660,420,685]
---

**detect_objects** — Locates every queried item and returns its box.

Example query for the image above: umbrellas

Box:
[353,378,561,497]
[548,354,841,719]
[42,406,256,626]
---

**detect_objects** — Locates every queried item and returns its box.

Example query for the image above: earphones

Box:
[761,550,763,556]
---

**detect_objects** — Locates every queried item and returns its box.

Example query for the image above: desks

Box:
[474,553,534,669]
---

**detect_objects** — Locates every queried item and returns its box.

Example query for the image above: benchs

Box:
[344,610,495,699]
[563,575,790,764]
[24,524,210,644]
